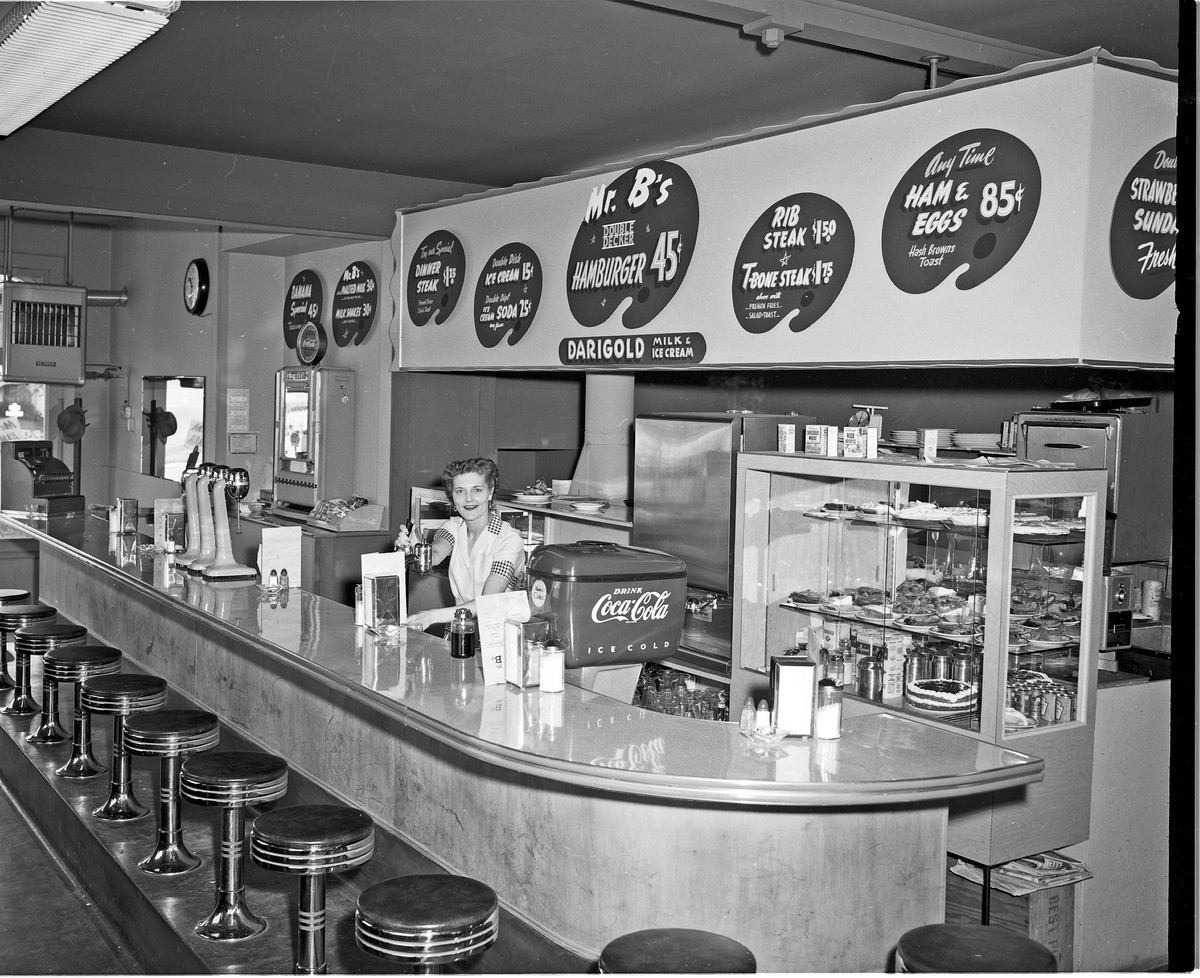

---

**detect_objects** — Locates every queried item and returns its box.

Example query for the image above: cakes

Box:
[904,678,978,712]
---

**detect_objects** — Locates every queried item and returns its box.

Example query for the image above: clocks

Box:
[183,258,209,317]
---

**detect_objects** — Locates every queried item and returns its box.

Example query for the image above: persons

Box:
[394,458,526,632]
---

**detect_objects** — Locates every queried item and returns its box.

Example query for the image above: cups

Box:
[1142,580,1164,621]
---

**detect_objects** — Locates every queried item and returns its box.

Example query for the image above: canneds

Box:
[1007,681,1075,727]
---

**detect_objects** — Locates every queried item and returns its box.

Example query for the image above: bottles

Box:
[279,590,289,609]
[756,700,771,735]
[449,608,476,659]
[280,569,289,590]
[354,584,366,626]
[837,638,853,685]
[268,592,278,609]
[827,654,844,691]
[904,642,973,685]
[856,657,879,702]
[739,697,757,735]
[538,638,566,693]
[268,569,278,592]
[813,678,842,740]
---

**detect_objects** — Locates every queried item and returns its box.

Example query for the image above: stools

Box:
[884,924,1058,973]
[80,673,169,822]
[121,709,220,876]
[597,928,757,974]
[356,873,499,974]
[0,588,87,745]
[43,645,122,779]
[250,804,375,975]
[179,752,288,942]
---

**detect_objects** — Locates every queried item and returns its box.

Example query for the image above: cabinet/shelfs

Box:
[729,452,1108,926]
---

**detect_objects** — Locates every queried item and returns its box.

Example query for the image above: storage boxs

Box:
[525,540,689,669]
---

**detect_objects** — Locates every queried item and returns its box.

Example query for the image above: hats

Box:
[156,407,177,445]
[57,404,85,444]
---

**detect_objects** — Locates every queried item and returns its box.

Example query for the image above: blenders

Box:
[173,460,257,580]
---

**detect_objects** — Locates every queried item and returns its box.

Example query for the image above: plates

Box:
[889,427,1001,448]
[570,503,605,511]
[511,492,554,502]
[808,508,1087,536]
[787,595,1081,651]
